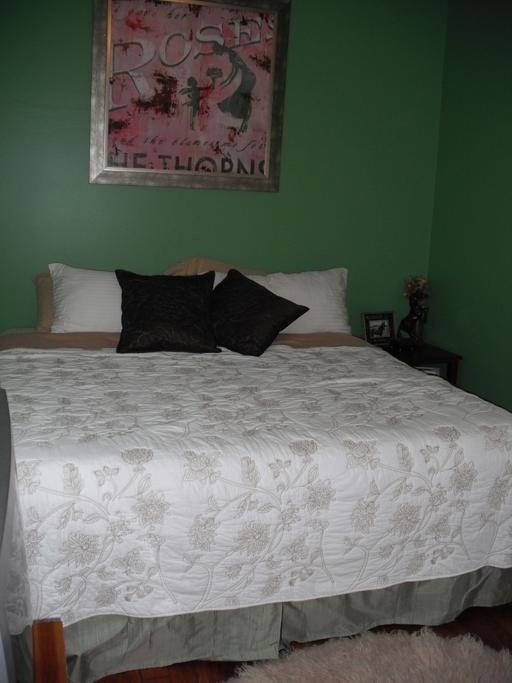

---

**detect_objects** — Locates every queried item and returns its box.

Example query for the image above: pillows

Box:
[209,268,310,357]
[33,272,56,333]
[112,266,222,354]
[205,268,351,335]
[48,264,123,333]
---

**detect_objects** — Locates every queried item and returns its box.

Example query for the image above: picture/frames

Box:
[86,0,291,193]
[360,311,397,345]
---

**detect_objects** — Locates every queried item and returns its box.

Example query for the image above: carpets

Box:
[222,626,512,682]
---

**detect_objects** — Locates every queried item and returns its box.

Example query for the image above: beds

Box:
[1,323,512,681]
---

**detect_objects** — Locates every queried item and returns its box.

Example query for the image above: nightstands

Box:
[380,341,463,386]
